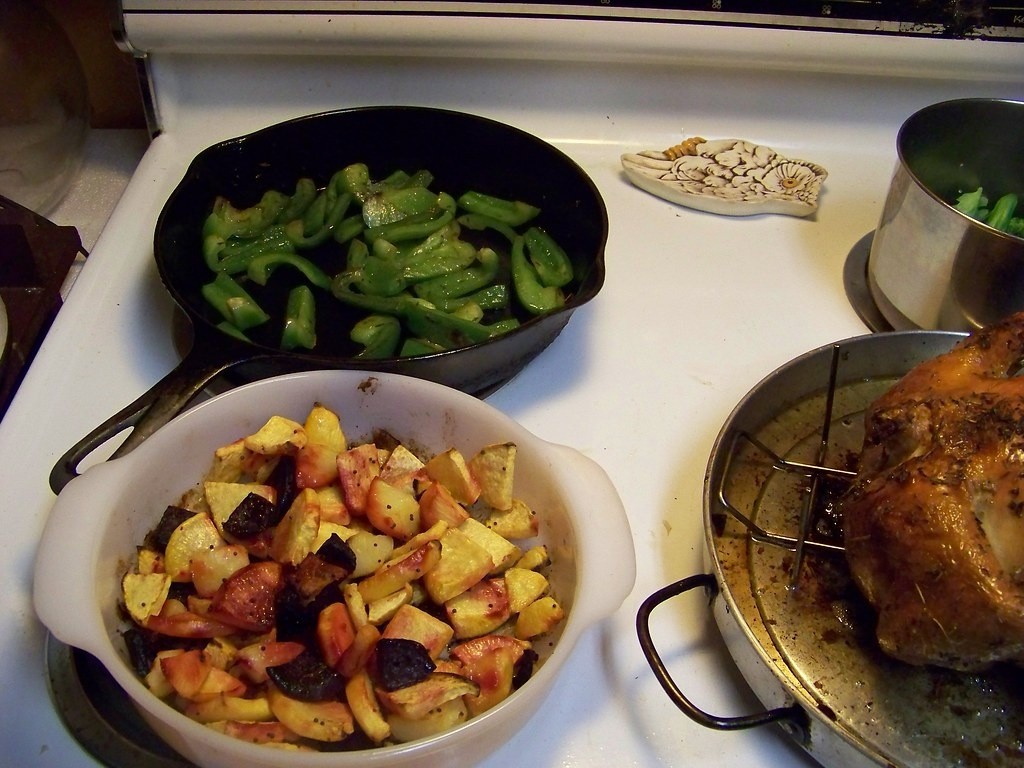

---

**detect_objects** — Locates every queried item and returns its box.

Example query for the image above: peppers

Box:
[202,162,574,357]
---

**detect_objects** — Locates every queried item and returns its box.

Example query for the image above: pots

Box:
[636,328,1024,768]
[48,106,609,496]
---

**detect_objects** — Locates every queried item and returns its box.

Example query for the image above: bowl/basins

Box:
[32,369,636,767]
[868,97,1024,332]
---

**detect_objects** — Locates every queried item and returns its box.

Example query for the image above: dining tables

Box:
[0,136,896,768]
